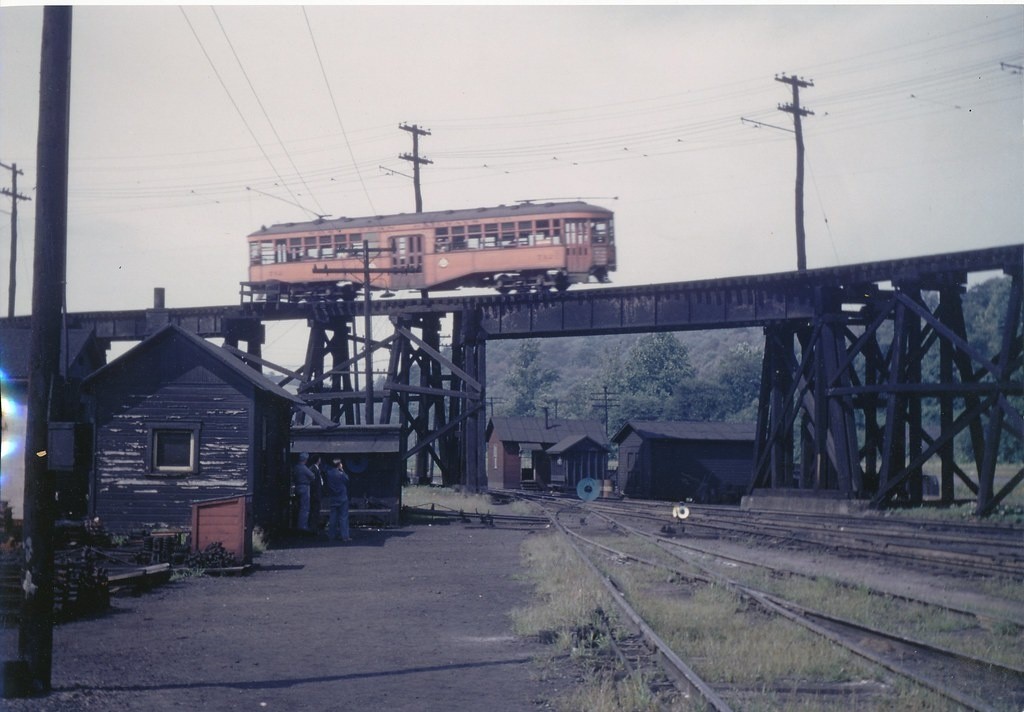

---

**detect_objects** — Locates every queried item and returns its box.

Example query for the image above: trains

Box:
[246,186,618,303]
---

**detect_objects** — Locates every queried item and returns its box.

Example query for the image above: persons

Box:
[309,454,325,535]
[293,452,315,535]
[327,458,352,542]
[592,222,598,243]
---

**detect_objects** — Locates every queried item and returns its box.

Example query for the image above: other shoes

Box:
[342,538,353,542]
